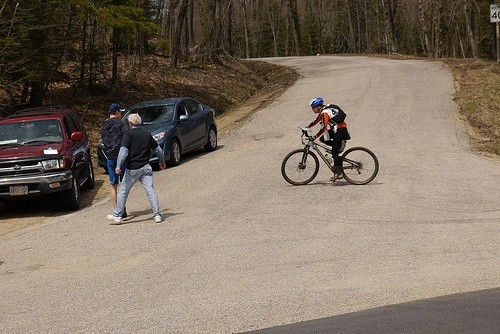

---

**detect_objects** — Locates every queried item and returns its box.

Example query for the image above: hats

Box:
[109,103,125,112]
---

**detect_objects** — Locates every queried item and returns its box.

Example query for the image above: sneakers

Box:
[154,214,162,223]
[106,214,122,222]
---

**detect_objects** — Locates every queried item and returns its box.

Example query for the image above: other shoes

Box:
[330,173,344,182]
[121,207,127,218]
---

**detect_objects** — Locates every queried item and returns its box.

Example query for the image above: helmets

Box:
[310,97,324,107]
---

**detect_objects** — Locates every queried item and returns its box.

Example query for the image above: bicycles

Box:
[281,126,380,186]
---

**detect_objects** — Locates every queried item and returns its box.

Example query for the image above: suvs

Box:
[0,107,96,217]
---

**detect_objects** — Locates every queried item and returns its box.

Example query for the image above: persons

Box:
[101,104,129,217]
[302,97,350,181]
[107,114,166,223]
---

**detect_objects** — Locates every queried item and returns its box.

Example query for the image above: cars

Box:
[97,97,218,174]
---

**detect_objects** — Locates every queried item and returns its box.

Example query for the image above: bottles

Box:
[339,140,347,151]
[325,154,334,166]
